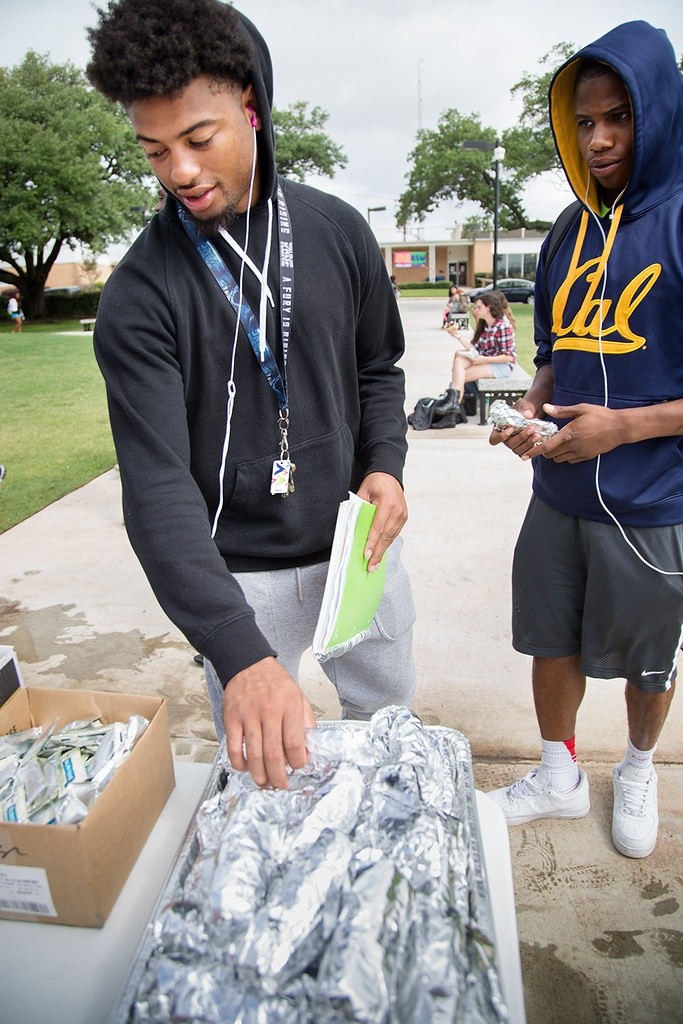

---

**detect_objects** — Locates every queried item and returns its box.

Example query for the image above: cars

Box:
[467,279,535,304]
[44,285,81,294]
[1,286,50,299]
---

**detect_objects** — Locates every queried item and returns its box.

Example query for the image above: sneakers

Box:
[486,763,590,826]
[611,760,659,860]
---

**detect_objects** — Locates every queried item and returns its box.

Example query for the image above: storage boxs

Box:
[0,686,177,930]
[0,644,26,709]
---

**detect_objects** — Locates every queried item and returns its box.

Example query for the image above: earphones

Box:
[247,104,258,127]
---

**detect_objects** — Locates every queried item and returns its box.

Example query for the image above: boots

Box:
[435,388,461,414]
[431,413,455,430]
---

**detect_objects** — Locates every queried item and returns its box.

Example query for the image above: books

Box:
[311,490,390,663]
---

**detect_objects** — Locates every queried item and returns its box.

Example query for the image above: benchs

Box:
[79,319,97,331]
[449,311,470,330]
[463,361,534,426]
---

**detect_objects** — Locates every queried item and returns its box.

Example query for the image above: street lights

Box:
[461,139,506,291]
[367,207,387,224]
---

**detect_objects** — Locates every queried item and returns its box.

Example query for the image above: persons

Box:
[481,16,680,860]
[389,274,401,303]
[431,286,518,431]
[6,291,25,335]
[86,0,418,798]
